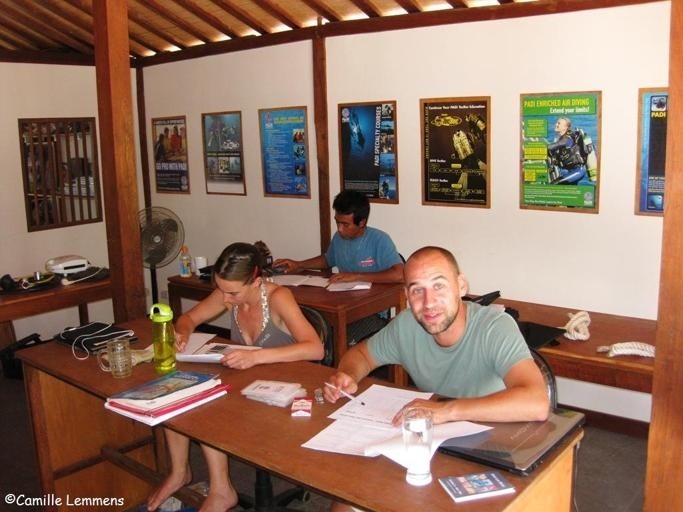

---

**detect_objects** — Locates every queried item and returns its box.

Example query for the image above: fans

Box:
[135,205,184,306]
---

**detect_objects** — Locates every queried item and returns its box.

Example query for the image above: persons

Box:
[144,241,327,512]
[270,189,406,284]
[323,244,551,512]
[155,125,182,160]
[547,115,589,184]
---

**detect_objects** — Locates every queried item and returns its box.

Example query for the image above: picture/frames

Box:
[634,87,668,217]
[151,115,191,195]
[200,111,247,195]
[519,89,600,215]
[337,100,400,204]
[256,106,312,200]
[418,95,492,208]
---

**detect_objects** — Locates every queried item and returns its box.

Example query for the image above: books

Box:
[436,467,518,505]
[101,368,233,428]
[238,379,307,409]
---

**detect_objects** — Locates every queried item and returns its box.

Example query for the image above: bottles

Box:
[148,304,176,372]
[178,246,191,278]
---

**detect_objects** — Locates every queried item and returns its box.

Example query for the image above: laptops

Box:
[437,407,587,477]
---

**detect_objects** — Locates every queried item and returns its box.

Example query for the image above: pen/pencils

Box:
[324,382,365,407]
[171,324,183,353]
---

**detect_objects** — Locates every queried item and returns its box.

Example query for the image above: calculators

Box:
[206,346,229,354]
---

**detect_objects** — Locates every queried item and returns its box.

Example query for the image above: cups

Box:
[401,406,432,487]
[193,256,207,277]
[33,271,42,281]
[96,338,132,379]
